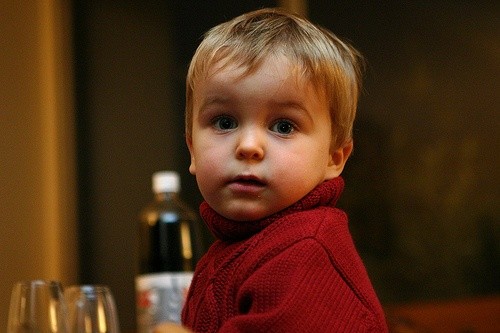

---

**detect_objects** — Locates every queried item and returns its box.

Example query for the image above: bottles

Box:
[135,171,198,332]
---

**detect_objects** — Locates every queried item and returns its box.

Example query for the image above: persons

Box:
[153,9,388,333]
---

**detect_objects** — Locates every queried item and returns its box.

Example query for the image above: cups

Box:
[60,285,120,333]
[6,281,70,333]
